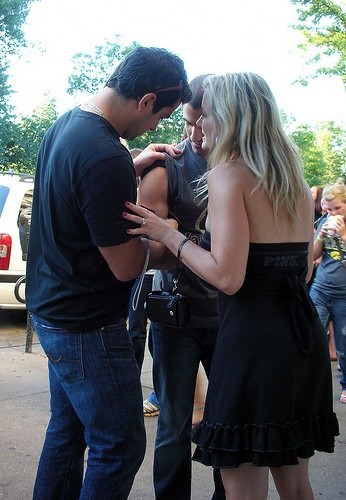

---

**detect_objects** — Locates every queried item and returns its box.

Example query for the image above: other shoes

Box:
[340,390,346,403]
[142,398,160,417]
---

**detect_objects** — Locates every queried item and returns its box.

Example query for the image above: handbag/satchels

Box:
[147,238,191,330]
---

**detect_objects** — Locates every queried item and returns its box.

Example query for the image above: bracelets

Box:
[317,235,324,241]
[177,237,190,261]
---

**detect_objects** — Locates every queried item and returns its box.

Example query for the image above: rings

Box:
[140,218,146,225]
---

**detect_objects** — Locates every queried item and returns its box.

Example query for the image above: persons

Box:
[305,183,346,404]
[127,148,162,415]
[191,360,209,427]
[122,72,341,500]
[140,72,226,500]
[26,44,191,500]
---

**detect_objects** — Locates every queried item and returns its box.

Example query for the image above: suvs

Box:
[0,171,34,310]
